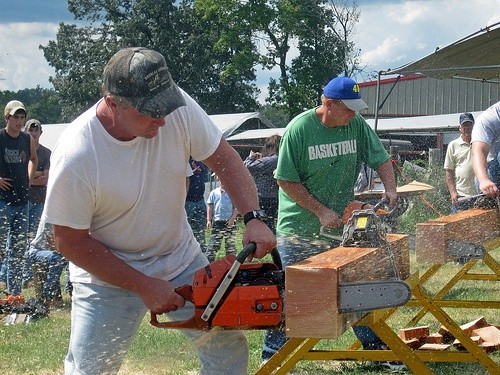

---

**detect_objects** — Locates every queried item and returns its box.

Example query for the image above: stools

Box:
[32,264,62,305]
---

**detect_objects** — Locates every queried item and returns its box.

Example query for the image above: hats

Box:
[103,47,187,118]
[324,75,368,110]
[25,119,42,132]
[4,100,27,119]
[460,113,474,125]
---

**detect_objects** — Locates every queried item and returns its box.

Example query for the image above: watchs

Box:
[244,209,268,226]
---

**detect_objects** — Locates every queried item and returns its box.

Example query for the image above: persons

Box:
[260,77,407,371]
[0,100,500,298]
[40,47,278,375]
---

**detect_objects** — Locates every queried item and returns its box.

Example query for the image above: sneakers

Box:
[365,345,408,373]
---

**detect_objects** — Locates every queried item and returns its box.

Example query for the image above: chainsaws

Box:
[149,238,412,333]
[318,195,487,262]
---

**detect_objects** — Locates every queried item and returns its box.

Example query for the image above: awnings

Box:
[369,23,500,191]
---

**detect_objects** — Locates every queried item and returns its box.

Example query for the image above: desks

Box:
[354,180,436,218]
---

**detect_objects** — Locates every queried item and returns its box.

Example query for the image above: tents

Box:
[22,112,277,193]
[226,111,486,146]
